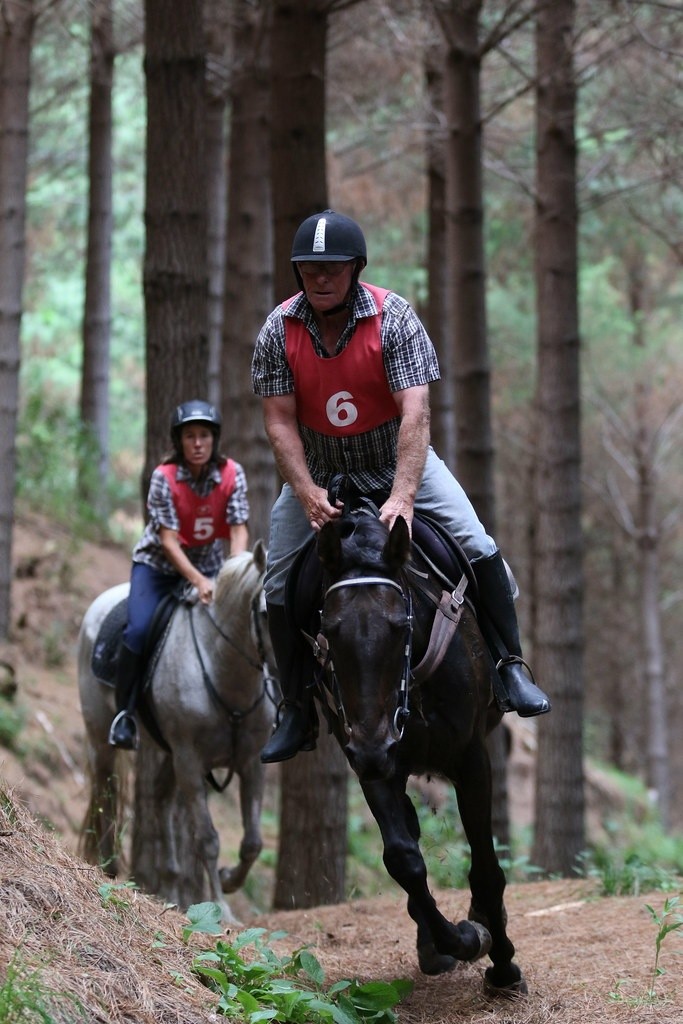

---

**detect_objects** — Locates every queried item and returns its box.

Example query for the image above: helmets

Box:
[168,400,223,451]
[290,209,367,270]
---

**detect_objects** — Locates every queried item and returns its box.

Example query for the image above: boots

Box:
[469,549,552,718]
[261,604,320,763]
[108,646,156,750]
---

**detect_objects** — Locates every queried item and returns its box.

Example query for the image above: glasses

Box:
[300,261,350,277]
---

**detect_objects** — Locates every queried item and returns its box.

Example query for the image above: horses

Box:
[75,537,282,930]
[289,496,528,1000]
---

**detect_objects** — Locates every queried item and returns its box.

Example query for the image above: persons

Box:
[251,209,552,763]
[108,402,250,751]
